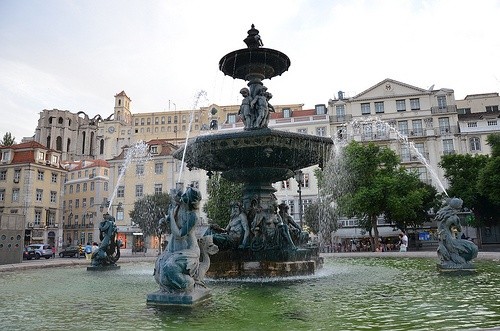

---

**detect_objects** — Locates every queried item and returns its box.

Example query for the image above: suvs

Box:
[25,243,53,260]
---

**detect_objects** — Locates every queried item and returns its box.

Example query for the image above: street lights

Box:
[294,170,303,231]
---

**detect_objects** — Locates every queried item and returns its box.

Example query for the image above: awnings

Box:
[133,233,144,235]
[334,226,402,238]
[117,225,144,232]
[32,236,45,240]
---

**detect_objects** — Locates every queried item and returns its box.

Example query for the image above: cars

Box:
[59,246,85,258]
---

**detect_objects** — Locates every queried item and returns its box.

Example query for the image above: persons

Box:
[396,230,408,252]
[247,24,259,38]
[141,239,146,252]
[254,35,263,46]
[437,197,468,259]
[79,244,82,254]
[84,242,99,259]
[91,213,119,265]
[51,243,55,258]
[153,186,202,291]
[237,86,276,130]
[461,233,465,239]
[320,239,394,253]
[204,200,309,261]
